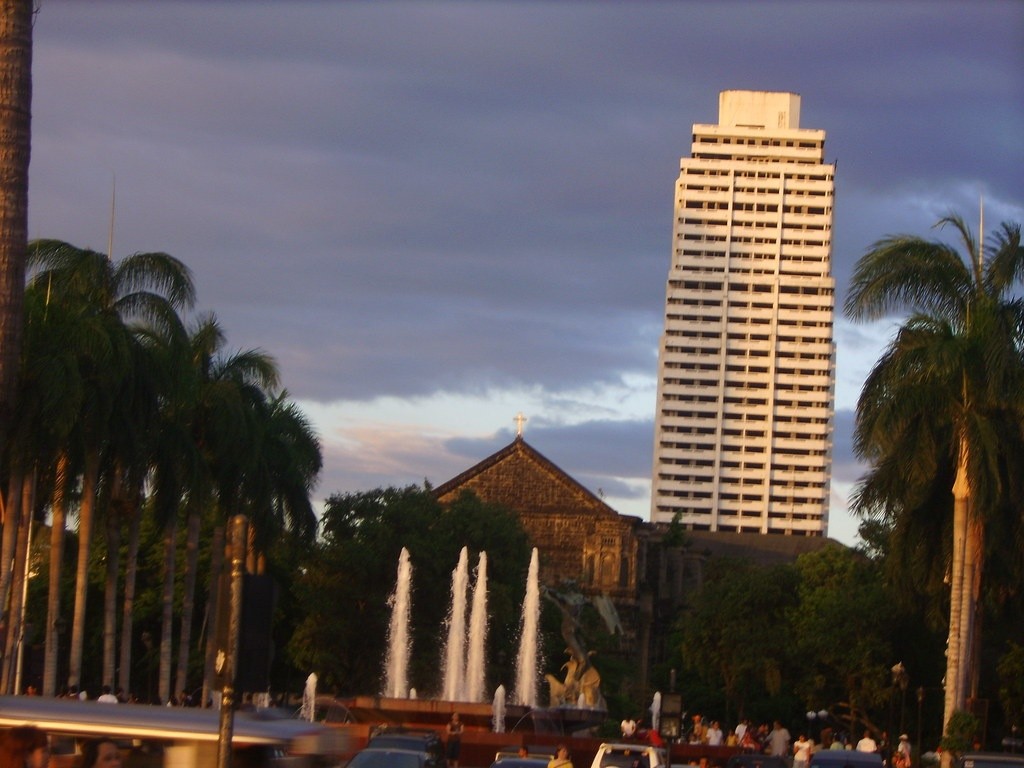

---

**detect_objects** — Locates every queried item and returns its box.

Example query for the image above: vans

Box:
[0,691,353,767]
[592,741,667,768]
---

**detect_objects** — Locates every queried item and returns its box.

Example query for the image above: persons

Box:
[518,700,981,768]
[0,681,301,768]
[446,712,463,767]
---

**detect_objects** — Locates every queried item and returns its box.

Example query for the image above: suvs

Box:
[365,724,447,768]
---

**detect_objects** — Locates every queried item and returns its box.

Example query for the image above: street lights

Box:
[898,670,910,742]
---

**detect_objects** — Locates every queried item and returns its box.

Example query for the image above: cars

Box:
[342,747,438,768]
[489,743,557,768]
[807,750,885,768]
